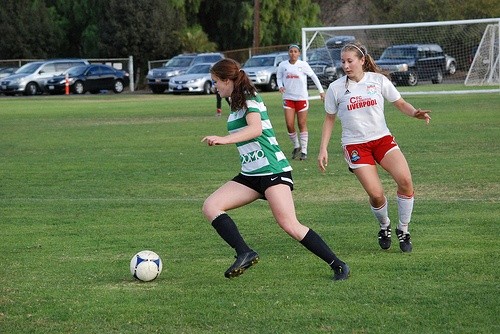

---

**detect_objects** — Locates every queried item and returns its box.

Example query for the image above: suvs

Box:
[239,52,302,92]
[306,36,355,90]
[372,43,447,87]
[145,52,226,95]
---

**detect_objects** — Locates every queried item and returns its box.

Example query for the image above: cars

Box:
[168,62,217,95]
[45,63,129,96]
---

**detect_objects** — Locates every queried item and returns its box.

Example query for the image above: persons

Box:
[318,41,432,253]
[211,78,231,114]
[200,59,351,282]
[276,45,325,161]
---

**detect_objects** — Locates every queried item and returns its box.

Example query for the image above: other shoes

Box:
[216,113,221,117]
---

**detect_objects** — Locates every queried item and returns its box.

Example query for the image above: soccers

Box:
[129,250,163,282]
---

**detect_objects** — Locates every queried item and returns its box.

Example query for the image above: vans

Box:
[0,59,90,97]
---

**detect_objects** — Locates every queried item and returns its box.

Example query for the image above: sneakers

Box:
[395,224,413,252]
[377,219,391,250]
[300,152,307,160]
[292,147,301,159]
[331,261,350,280]
[224,249,259,278]
